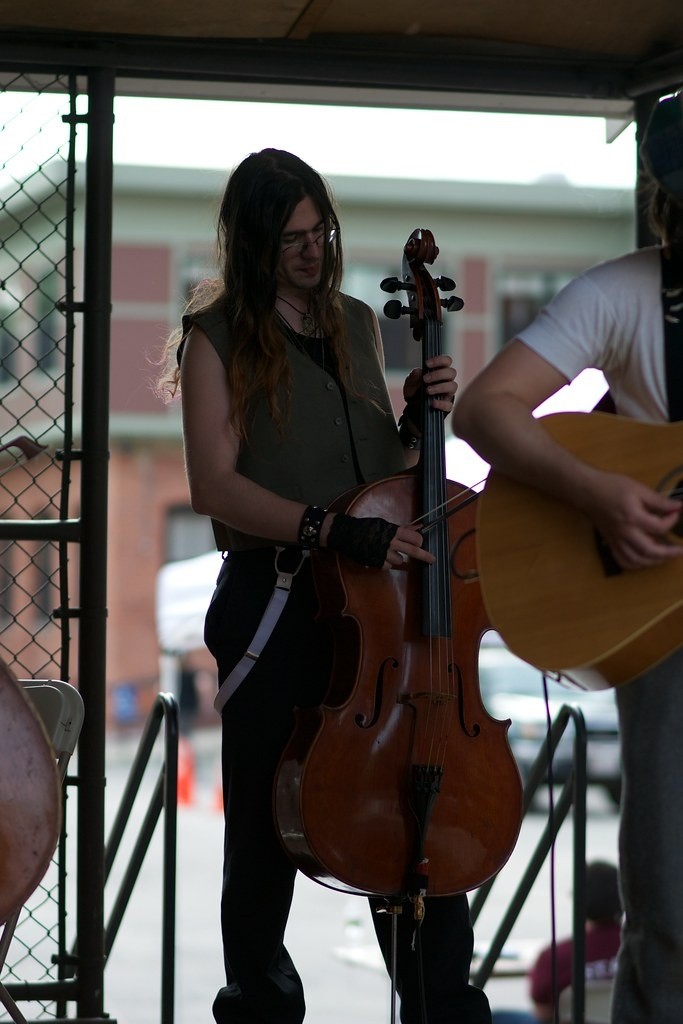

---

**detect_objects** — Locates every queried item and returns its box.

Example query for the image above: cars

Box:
[477,646,622,812]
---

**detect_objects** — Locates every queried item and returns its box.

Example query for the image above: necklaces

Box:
[273,293,318,337]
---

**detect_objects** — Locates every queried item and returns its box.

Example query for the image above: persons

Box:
[452,83,682,1024]
[485,861,625,1024]
[149,145,496,1024]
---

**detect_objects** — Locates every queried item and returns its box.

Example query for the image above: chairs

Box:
[1,678,84,1024]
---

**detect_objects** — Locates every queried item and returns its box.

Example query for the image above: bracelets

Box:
[296,499,329,559]
[396,414,424,451]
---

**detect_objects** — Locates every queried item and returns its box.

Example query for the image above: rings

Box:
[449,401,453,412]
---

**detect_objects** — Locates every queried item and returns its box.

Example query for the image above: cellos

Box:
[270,227,524,1024]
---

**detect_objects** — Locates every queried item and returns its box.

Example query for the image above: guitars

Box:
[475,410,683,693]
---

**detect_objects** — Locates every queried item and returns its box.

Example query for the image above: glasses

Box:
[275,215,338,258]
